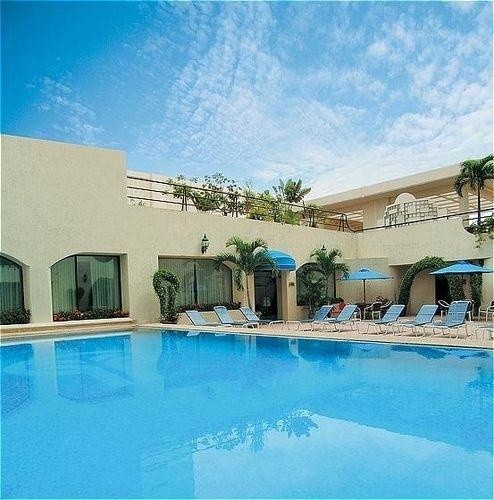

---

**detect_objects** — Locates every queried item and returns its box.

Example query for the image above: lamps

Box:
[200,234,209,254]
[321,245,327,254]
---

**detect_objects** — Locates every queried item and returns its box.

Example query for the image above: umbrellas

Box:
[337,267,394,320]
[428,260,493,300]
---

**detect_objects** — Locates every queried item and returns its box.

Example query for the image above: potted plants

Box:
[213,236,280,328]
[298,249,350,318]
[152,269,180,324]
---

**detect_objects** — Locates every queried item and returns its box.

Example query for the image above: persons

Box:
[338,297,346,313]
